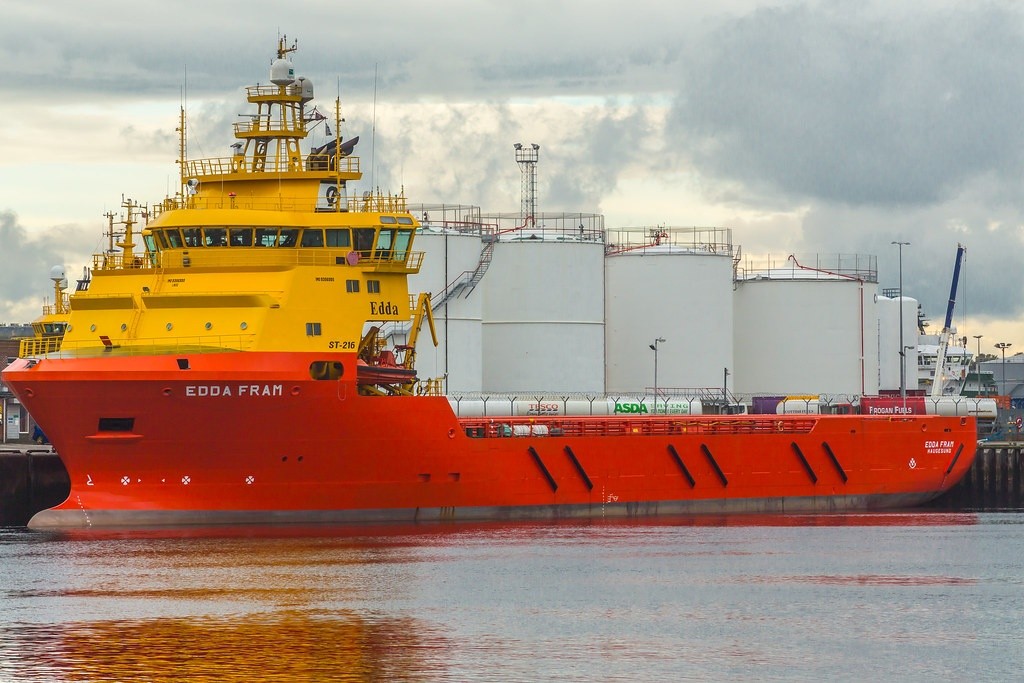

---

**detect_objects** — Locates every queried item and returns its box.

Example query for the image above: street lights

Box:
[995,342,1013,408]
[891,241,910,387]
[649,338,666,414]
[902,344,914,420]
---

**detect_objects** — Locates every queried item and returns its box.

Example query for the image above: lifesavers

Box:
[417,385,423,394]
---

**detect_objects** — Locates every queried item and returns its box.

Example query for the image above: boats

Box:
[0,31,978,535]
